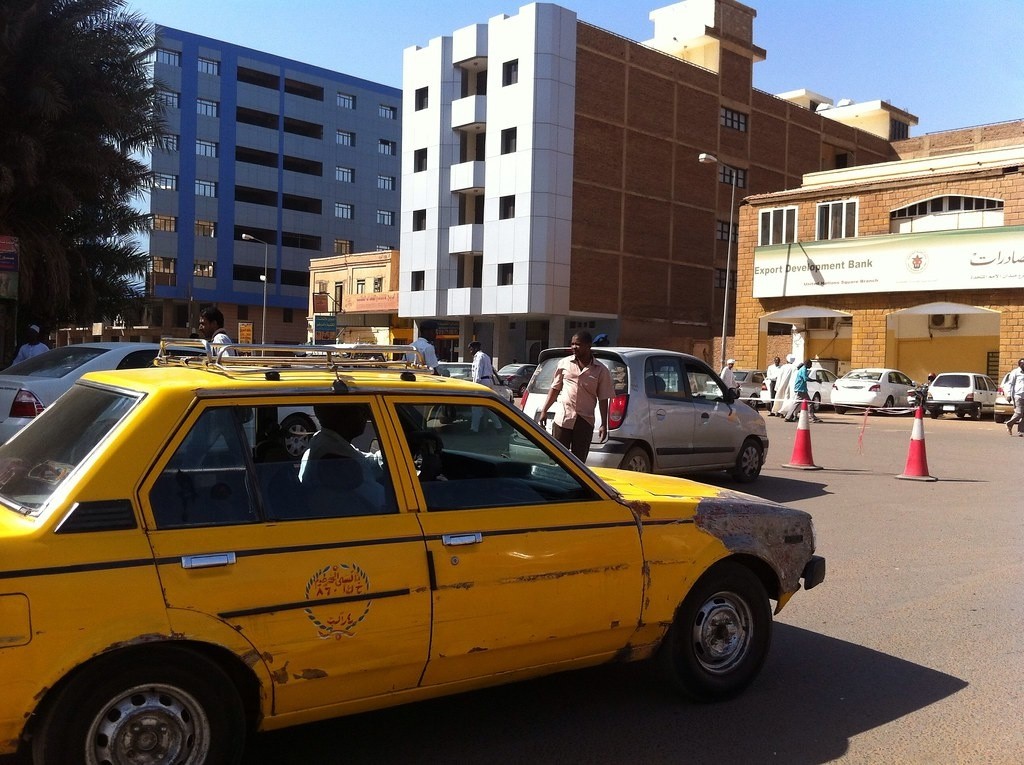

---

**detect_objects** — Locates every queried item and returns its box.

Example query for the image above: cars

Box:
[1,343,319,463]
[760,367,838,412]
[830,368,917,415]
[993,372,1016,424]
[508,346,769,486]
[705,368,768,409]
[494,364,538,397]
[436,361,516,410]
[1,338,828,765]
[925,372,997,422]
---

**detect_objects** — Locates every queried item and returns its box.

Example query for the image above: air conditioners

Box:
[927,314,958,329]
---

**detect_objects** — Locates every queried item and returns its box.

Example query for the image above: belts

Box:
[480,376,493,379]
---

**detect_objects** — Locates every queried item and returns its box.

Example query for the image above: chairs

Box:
[646,375,667,396]
[281,454,375,511]
[245,439,295,517]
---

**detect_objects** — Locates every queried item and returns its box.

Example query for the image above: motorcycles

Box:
[906,379,931,416]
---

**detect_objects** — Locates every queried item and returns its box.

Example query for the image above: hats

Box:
[786,354,795,363]
[592,333,607,344]
[468,341,481,348]
[30,324,40,333]
[420,320,440,331]
[727,359,735,365]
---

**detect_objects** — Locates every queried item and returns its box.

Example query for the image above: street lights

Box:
[242,234,268,356]
[698,152,738,374]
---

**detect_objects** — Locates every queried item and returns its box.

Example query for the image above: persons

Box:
[298,403,443,516]
[767,353,824,424]
[1006,359,1024,436]
[402,321,450,378]
[10,325,50,366]
[178,307,254,469]
[717,359,740,396]
[466,340,503,433]
[538,331,617,465]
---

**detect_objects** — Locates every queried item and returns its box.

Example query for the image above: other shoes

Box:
[784,418,795,422]
[767,413,784,418]
[1006,423,1012,435]
[1018,431,1024,437]
[809,418,823,423]
[462,430,478,436]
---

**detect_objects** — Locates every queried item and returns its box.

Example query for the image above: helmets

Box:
[928,373,936,380]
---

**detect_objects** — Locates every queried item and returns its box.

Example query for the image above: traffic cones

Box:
[894,406,939,483]
[779,401,825,471]
